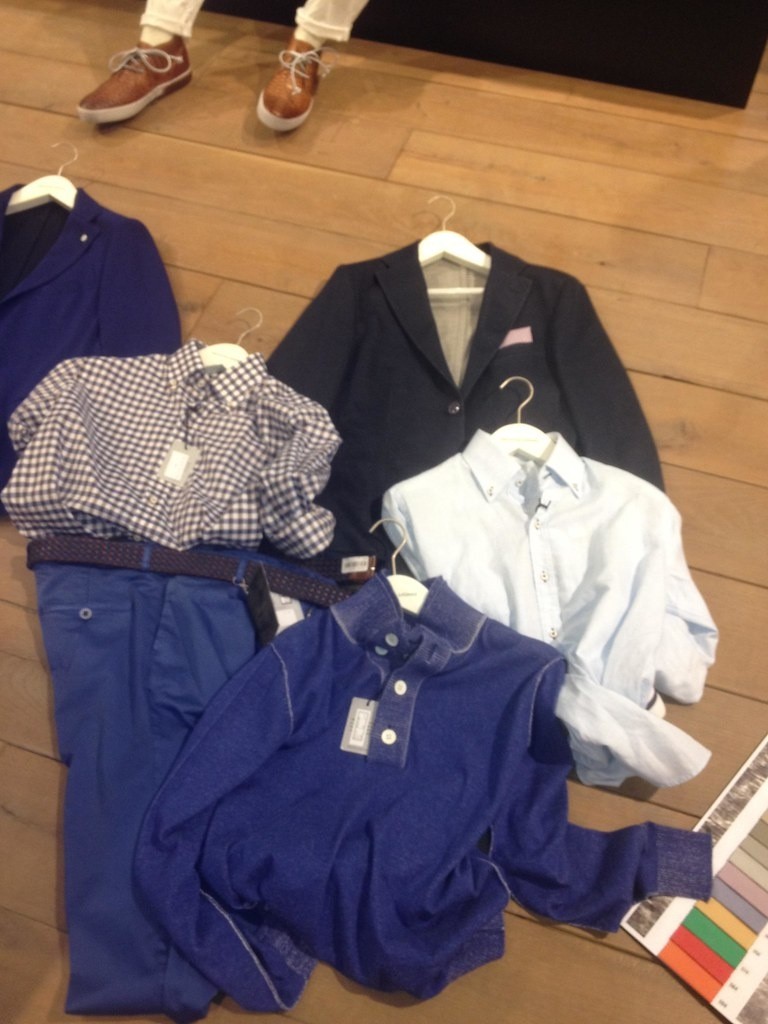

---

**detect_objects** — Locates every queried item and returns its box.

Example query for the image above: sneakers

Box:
[78,34,193,124]
[257,34,320,131]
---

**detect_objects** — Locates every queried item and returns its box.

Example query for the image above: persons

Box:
[76,1,375,132]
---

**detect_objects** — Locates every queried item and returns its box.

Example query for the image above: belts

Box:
[23,536,353,607]
